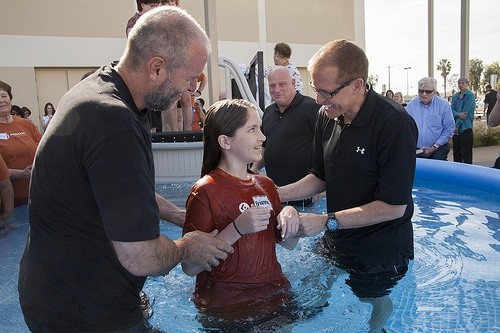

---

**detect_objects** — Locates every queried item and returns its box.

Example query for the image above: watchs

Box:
[324,212,339,232]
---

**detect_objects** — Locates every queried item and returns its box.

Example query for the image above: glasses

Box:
[308,80,352,98]
[418,89,434,94]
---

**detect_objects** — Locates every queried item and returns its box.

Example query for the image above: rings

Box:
[211,260,216,265]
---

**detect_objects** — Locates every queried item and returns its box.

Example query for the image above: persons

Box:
[483,84,497,128]
[182,100,300,308]
[250,66,325,207]
[81,0,206,133]
[275,38,418,268]
[452,78,475,164]
[405,77,455,160]
[18,5,235,333]
[239,42,303,105]
[488,84,500,170]
[0,81,56,220]
[381,90,452,110]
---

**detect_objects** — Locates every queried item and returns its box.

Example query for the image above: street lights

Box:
[404,67,411,99]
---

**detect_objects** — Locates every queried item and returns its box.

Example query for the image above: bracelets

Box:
[233,221,243,236]
[196,90,201,95]
[433,145,438,150]
